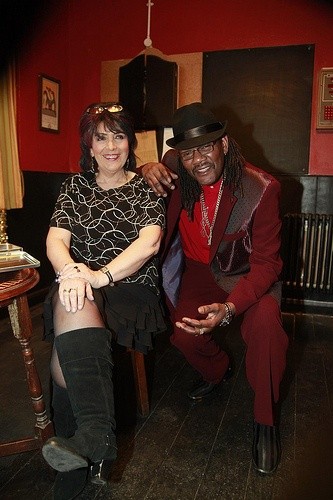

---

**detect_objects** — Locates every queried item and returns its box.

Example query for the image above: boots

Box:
[43,326,118,486]
[50,380,90,500]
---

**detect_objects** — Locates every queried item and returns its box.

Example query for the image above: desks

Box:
[0,266,59,485]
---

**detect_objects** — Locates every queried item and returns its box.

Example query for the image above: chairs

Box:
[43,277,150,420]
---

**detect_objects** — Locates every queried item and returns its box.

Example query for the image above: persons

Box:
[130,102,296,475]
[35,98,175,500]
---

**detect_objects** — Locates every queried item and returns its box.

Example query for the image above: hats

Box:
[166,102,228,150]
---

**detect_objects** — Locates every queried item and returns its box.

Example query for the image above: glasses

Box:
[177,140,218,161]
[85,103,126,115]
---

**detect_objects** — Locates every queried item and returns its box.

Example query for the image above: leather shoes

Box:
[186,364,234,400]
[249,417,282,478]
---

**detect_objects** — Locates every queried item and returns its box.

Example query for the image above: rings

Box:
[199,328,205,335]
[73,266,81,273]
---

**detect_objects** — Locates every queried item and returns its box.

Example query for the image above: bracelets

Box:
[221,301,233,327]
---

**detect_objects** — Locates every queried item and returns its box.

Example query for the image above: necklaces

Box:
[199,177,227,246]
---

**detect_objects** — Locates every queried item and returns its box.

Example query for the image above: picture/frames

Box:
[37,72,61,135]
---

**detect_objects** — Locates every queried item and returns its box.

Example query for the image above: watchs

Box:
[99,266,114,287]
